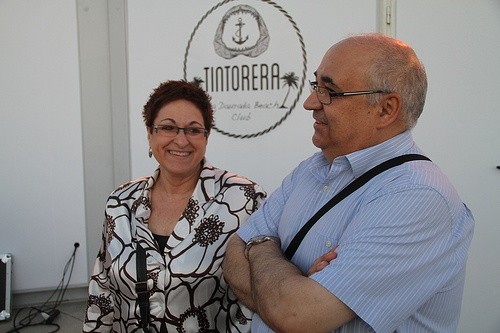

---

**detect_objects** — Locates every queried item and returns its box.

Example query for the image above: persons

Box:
[82,80,267,333]
[222,33,475,333]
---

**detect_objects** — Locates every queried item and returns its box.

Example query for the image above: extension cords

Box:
[45,309,60,324]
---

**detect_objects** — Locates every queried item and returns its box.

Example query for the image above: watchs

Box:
[244,235,278,258]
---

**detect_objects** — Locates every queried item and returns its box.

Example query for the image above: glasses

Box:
[152,125,208,137]
[308,81,393,104]
